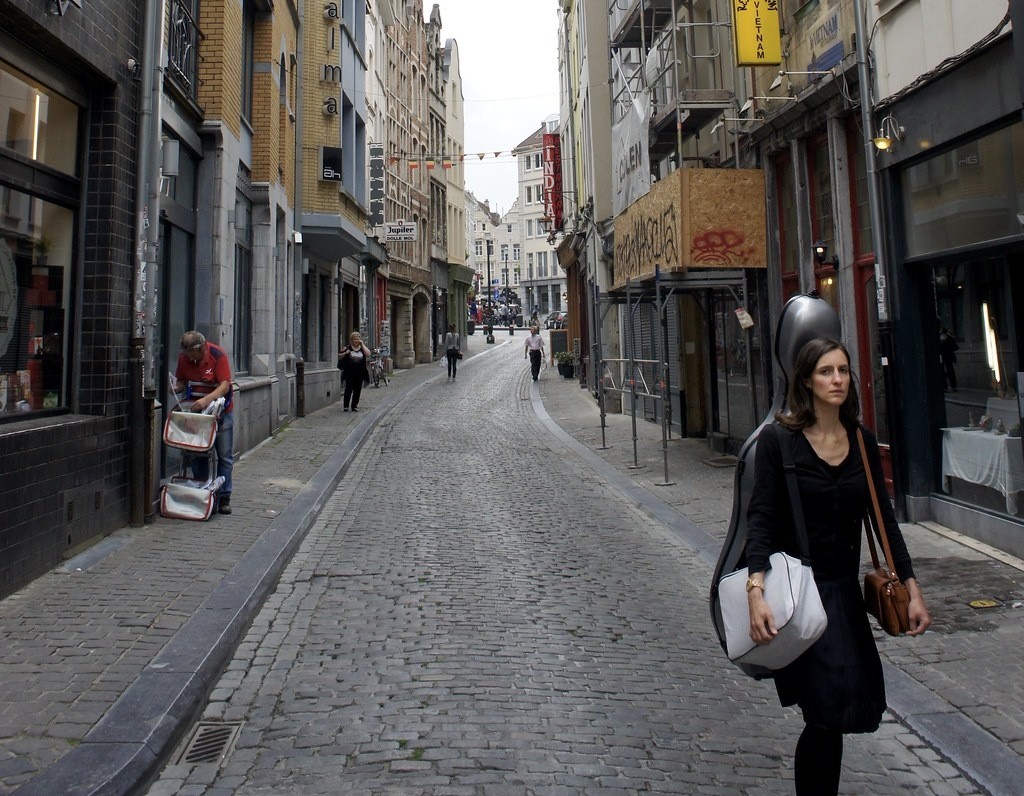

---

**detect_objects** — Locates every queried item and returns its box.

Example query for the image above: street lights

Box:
[504,248,508,308]
[529,258,533,314]
[485,232,495,344]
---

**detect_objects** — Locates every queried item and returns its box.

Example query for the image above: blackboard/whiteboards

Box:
[550,329,570,360]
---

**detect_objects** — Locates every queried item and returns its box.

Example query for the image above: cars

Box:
[544,311,568,329]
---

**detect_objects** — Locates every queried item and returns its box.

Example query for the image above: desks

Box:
[941,426,1024,515]
[986,397,1024,435]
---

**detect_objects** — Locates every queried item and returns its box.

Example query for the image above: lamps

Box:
[769,67,835,91]
[740,95,798,113]
[812,237,840,269]
[872,116,905,150]
[712,116,766,134]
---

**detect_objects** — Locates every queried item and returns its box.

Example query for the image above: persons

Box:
[940,328,959,393]
[337,332,371,411]
[442,323,461,382]
[166,331,234,515]
[746,337,931,796]
[466,299,543,327]
[524,326,546,381]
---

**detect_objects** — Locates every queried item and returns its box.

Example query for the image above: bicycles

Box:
[370,346,390,387]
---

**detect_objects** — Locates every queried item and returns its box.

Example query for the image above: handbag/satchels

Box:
[541,357,547,370]
[337,344,349,370]
[718,552,827,675]
[440,356,448,368]
[864,567,910,637]
[458,351,462,359]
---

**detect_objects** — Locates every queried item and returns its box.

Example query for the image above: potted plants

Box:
[554,350,576,378]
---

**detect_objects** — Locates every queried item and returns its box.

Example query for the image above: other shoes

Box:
[352,407,359,411]
[344,407,348,411]
[220,496,232,514]
[533,377,538,381]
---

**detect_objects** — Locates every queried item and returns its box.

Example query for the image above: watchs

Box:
[746,579,764,592]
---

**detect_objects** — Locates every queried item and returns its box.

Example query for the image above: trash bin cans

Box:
[467,320,475,335]
[516,315,523,327]
